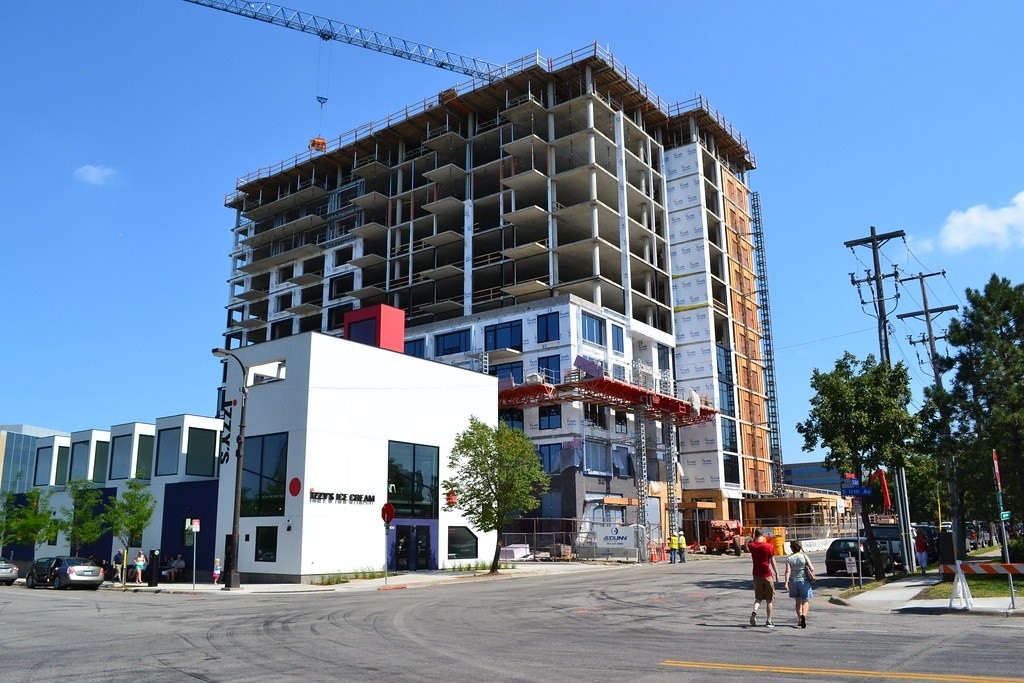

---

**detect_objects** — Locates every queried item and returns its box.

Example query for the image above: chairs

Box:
[841,542,850,549]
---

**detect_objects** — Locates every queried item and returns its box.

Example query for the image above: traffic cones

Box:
[651,547,659,563]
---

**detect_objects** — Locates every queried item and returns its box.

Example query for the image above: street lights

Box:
[211,348,249,591]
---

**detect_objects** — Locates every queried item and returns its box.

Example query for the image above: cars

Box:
[825,520,1024,577]
[26,555,104,591]
[0,557,19,586]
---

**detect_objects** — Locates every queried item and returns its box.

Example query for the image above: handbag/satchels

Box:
[802,553,816,583]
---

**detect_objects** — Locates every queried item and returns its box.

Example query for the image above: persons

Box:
[1005,522,1010,539]
[111,549,124,583]
[47,560,60,582]
[1015,520,1023,538]
[667,531,686,563]
[213,559,222,584]
[785,541,815,629]
[90,556,96,564]
[915,533,928,578]
[748,528,779,627]
[133,551,146,584]
[161,554,185,582]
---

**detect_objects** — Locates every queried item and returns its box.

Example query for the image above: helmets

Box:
[672,532,677,536]
[679,531,684,535]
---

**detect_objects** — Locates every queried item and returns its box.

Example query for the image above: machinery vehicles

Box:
[705,520,753,556]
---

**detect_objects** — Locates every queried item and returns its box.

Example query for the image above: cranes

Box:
[180,1,523,85]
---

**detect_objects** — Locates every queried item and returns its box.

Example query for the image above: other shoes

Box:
[800,615,806,629]
[679,561,683,563]
[668,562,672,564]
[750,611,756,626]
[798,622,801,626]
[766,621,774,627]
